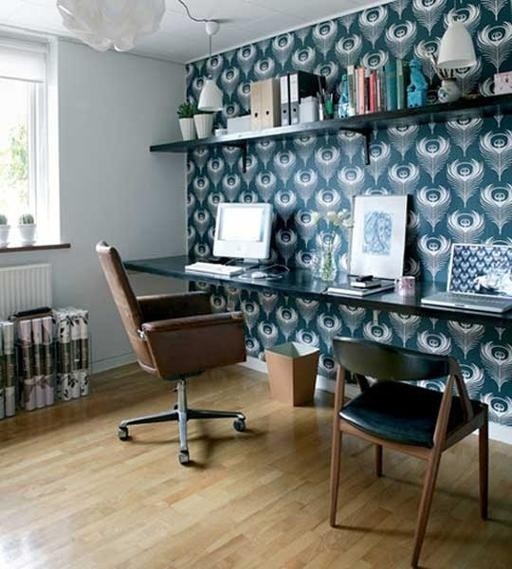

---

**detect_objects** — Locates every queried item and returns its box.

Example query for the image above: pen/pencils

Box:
[316,90,334,101]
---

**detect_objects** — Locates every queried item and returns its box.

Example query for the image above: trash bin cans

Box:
[264,341,320,408]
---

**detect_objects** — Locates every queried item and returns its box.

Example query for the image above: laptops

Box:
[420,243,512,314]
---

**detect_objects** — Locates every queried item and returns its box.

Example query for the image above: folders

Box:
[251,69,326,131]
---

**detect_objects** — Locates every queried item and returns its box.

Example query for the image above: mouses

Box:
[250,271,268,279]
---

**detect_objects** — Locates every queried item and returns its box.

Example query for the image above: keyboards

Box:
[184,260,243,278]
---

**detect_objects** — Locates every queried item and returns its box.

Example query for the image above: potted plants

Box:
[17,214,36,242]
[0,214,11,242]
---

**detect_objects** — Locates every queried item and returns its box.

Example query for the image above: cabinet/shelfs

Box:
[121,92,512,328]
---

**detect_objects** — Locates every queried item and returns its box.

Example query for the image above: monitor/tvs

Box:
[212,202,273,271]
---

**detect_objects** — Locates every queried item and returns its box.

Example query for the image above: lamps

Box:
[56,0,167,52]
[437,16,477,70]
[177,0,223,112]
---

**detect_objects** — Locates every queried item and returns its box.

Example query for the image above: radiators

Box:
[1,264,50,320]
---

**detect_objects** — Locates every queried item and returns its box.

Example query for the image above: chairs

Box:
[330,335,488,569]
[96,244,245,466]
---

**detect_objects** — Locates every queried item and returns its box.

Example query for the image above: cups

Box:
[395,276,415,297]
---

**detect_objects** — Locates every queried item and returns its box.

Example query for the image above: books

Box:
[251,56,411,133]
[351,279,383,288]
[327,281,395,299]
